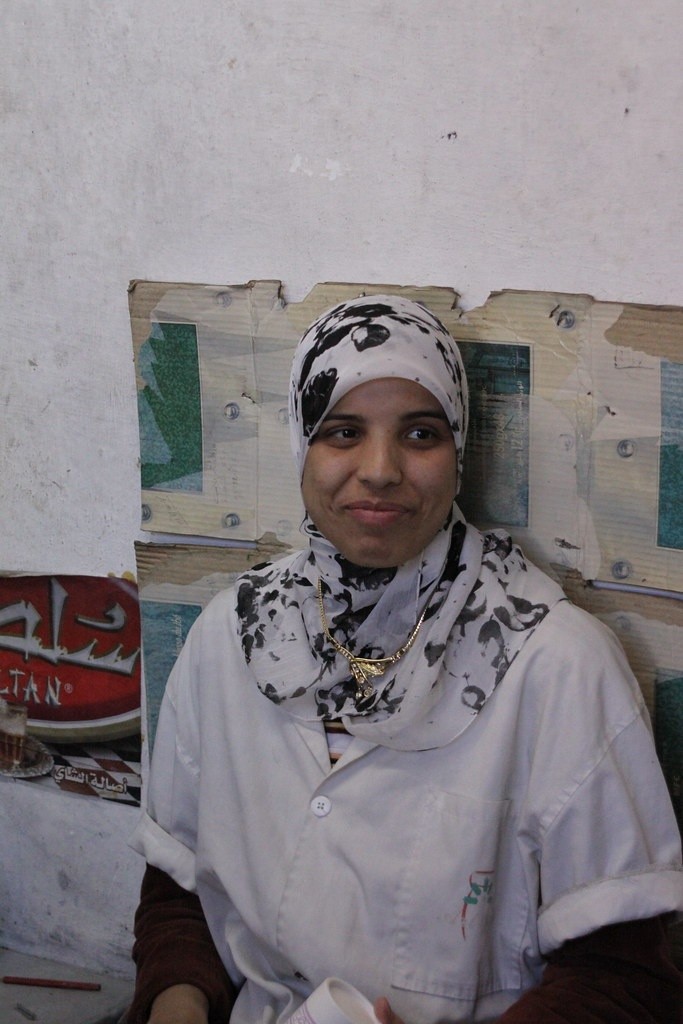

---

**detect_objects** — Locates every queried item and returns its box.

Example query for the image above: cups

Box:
[0,706,28,770]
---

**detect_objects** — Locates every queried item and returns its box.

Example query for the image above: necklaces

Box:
[317,580,437,699]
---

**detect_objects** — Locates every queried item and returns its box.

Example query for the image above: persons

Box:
[130,293,683,1024]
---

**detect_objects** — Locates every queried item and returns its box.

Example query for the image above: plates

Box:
[0,734,55,778]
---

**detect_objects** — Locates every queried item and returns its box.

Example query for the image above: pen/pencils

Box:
[2,976,101,992]
